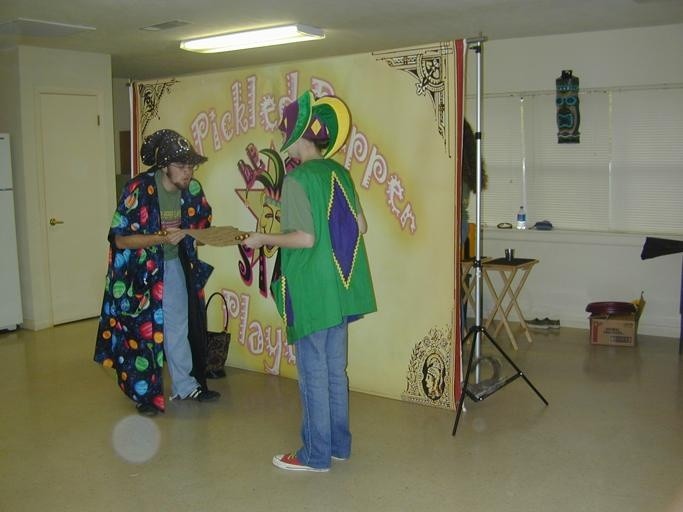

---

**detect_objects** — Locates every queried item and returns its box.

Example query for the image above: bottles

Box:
[505,248,513,263]
[516,205,527,230]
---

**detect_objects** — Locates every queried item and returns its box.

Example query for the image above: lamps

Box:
[179,24,326,54]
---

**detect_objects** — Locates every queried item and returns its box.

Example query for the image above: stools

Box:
[462,258,491,314]
[482,258,539,352]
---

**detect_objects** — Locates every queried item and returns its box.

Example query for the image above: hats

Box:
[279,99,332,142]
[138,127,207,174]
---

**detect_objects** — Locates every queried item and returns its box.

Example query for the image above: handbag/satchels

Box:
[194,290,232,380]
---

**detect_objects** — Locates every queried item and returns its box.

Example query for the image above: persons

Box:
[92,126,223,418]
[235,93,378,475]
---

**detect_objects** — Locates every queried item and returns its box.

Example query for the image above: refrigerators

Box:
[0,132,25,332]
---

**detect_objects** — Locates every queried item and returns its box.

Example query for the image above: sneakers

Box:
[167,386,220,402]
[270,445,347,477]
[540,317,562,329]
[521,317,549,329]
[134,398,157,418]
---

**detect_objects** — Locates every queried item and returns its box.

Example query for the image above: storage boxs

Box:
[590,291,647,348]
